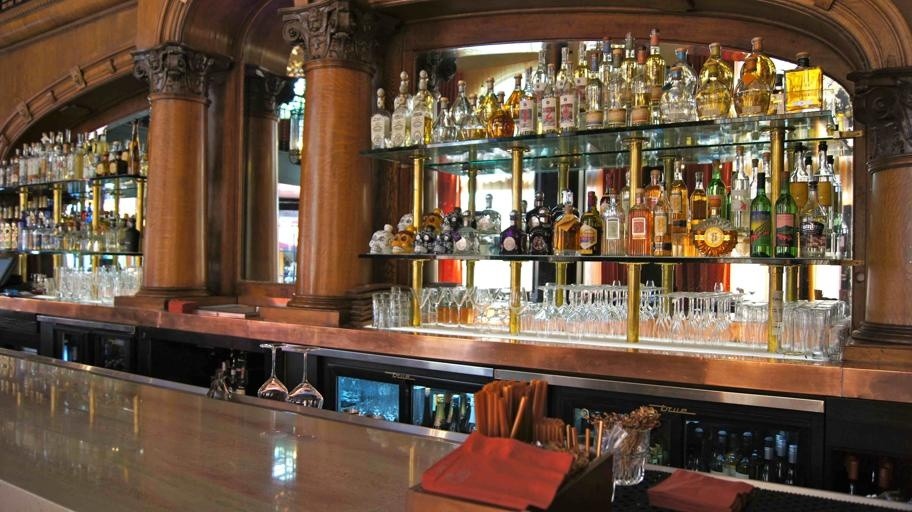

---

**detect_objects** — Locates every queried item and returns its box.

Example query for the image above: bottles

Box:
[217,348,248,395]
[841,455,896,500]
[0,117,148,253]
[365,24,848,261]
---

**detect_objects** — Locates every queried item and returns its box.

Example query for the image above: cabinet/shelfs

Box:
[2,175,148,282]
[354,111,866,366]
[0,296,912,512]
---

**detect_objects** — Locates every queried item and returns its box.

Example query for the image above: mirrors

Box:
[237,0,310,283]
[10,92,148,283]
[411,38,854,319]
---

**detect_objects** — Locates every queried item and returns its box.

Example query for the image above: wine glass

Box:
[287,343,325,410]
[257,341,290,403]
[371,280,748,350]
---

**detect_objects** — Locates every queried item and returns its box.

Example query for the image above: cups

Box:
[736,299,848,365]
[30,264,142,305]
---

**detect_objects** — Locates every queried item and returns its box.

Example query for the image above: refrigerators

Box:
[315,352,824,493]
